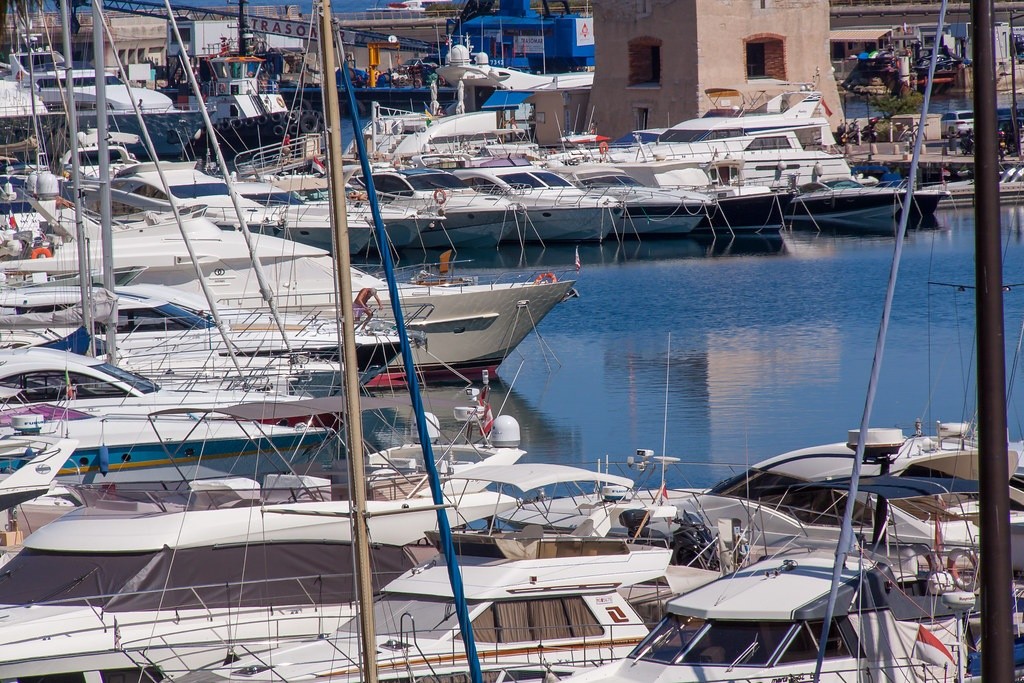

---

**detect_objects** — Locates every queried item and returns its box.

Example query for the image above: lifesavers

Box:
[29,248,52,260]
[276,97,285,108]
[532,272,558,287]
[945,543,980,593]
[597,140,609,154]
[432,188,448,207]
[217,83,226,94]
[208,109,325,137]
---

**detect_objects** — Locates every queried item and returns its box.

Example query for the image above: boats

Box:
[0,1,1024,683]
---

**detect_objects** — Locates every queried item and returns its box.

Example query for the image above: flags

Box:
[818,99,833,118]
[913,625,956,671]
[312,156,327,175]
[425,104,433,126]
[66,367,73,397]
[662,486,672,527]
[478,390,494,436]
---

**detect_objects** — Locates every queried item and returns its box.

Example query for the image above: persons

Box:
[906,47,913,68]
[352,288,384,333]
[283,134,292,165]
